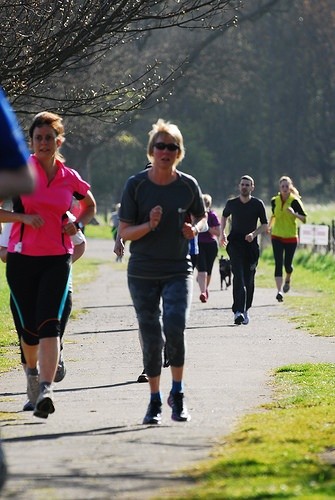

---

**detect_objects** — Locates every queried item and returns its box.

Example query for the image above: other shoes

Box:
[200,289,208,303]
[23,401,35,411]
[138,369,149,382]
[283,281,291,293]
[276,292,283,302]
[54,358,66,382]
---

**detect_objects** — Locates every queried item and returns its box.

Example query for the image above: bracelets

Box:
[148,221,155,232]
[193,224,199,236]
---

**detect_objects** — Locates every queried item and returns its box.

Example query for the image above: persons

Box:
[0,113,97,419]
[194,195,220,303]
[0,90,35,487]
[219,176,267,325]
[268,176,307,302]
[0,211,85,411]
[111,204,125,245]
[118,119,208,426]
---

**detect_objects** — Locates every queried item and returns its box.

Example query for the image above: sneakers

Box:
[25,369,39,404]
[34,385,55,419]
[143,399,162,424]
[168,392,187,421]
[234,312,249,324]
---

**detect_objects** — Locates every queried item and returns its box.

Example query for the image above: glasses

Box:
[153,141,180,152]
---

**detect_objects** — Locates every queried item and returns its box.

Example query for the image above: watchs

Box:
[74,221,83,229]
